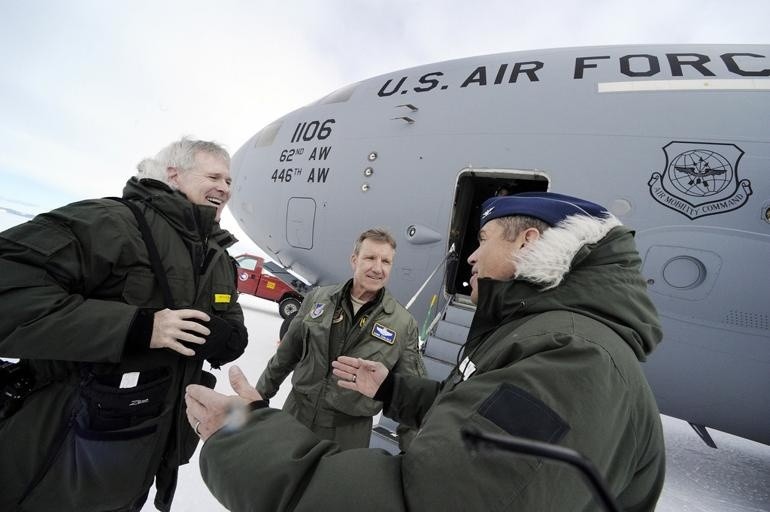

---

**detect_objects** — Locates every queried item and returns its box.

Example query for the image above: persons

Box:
[185,192,666,512]
[0,136,248,511]
[255,228,429,448]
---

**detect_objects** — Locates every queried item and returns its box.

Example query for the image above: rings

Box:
[194,420,201,436]
[352,376,356,382]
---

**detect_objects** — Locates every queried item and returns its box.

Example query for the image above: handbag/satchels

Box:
[0,357,36,422]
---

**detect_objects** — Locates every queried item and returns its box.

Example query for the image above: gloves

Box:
[195,315,249,368]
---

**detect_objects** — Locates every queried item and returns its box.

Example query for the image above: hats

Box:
[479,192,608,228]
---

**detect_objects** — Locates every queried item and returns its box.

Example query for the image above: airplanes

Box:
[226,40,770,457]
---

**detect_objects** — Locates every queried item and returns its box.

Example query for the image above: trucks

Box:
[231,251,305,321]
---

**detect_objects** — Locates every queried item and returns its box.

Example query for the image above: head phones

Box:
[494,184,504,197]
